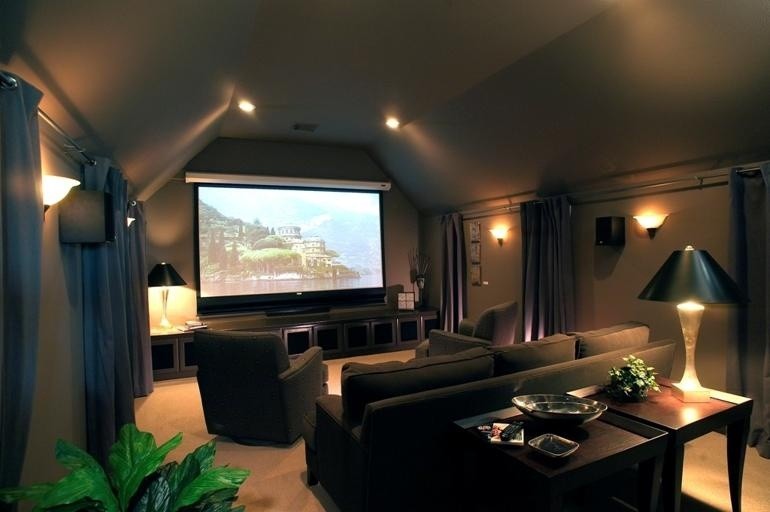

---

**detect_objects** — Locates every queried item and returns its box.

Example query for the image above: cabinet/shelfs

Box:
[283,319,340,360]
[148,331,198,381]
[341,311,398,356]
[396,304,441,351]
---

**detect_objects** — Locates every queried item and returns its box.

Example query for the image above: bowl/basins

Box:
[511,393,609,432]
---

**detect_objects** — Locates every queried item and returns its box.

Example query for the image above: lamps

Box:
[143,259,190,331]
[41,172,84,221]
[628,208,677,241]
[633,242,751,407]
[483,221,522,251]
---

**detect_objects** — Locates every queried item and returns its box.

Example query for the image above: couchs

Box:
[296,321,683,512]
[415,296,518,361]
[189,323,331,449]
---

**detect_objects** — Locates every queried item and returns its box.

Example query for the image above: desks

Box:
[450,393,671,512]
[564,368,757,510]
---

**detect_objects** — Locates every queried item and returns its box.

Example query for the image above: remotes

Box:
[502,421,523,440]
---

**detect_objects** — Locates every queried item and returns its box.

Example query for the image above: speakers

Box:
[595,215,624,245]
[59,191,114,244]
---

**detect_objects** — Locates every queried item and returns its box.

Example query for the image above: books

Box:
[490,421,525,446]
[179,321,207,332]
